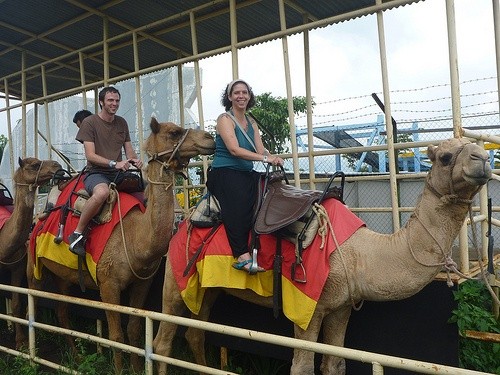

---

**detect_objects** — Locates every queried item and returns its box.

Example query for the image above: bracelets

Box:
[262,148,269,156]
[109,160,114,169]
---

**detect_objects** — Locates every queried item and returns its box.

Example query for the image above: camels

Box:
[0,156,65,350]
[152,138,492,375]
[27,117,216,375]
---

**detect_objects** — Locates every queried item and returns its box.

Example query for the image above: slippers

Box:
[232,259,266,272]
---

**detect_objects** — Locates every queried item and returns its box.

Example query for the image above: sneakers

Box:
[68,232,86,256]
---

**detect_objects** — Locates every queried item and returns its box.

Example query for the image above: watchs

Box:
[112,160,117,170]
[262,155,268,163]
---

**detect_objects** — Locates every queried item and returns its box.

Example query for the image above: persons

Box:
[73,110,94,129]
[68,86,144,258]
[207,78,285,272]
